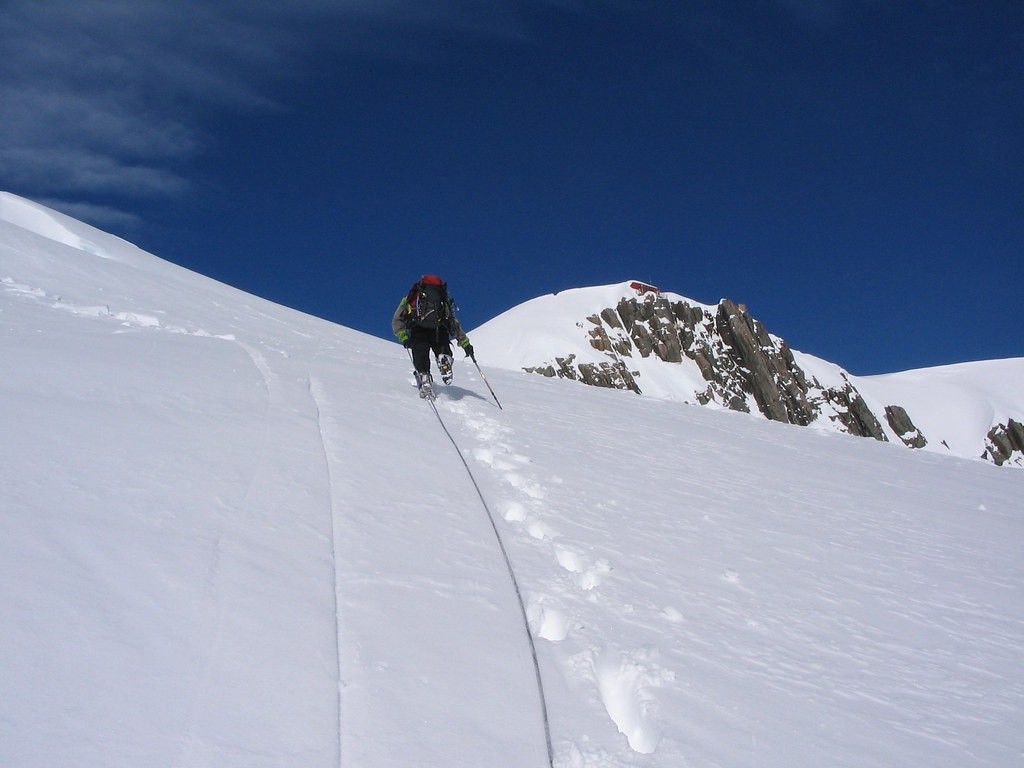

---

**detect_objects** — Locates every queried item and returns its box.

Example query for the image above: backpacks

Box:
[408,280,448,334]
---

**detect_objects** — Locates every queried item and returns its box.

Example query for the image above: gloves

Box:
[464,345,473,357]
[403,339,411,348]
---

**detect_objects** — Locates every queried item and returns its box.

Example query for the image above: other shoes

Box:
[419,385,432,397]
[438,354,453,385]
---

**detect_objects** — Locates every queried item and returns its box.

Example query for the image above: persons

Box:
[392,275,473,398]
